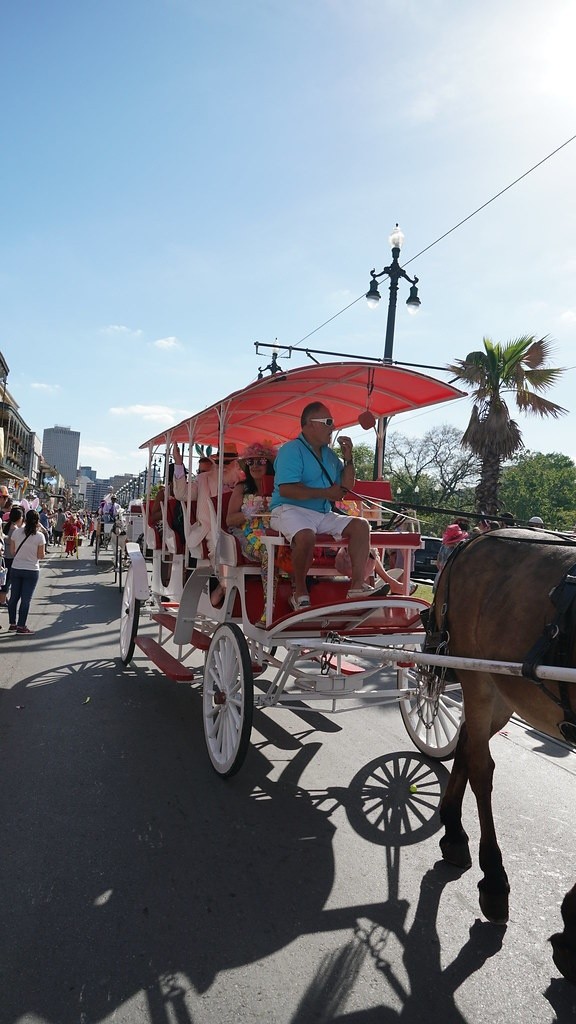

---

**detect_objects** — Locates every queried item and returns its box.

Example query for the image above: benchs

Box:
[131,491,374,617]
[250,474,425,632]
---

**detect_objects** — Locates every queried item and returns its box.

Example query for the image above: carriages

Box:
[91,495,133,569]
[118,359,576,930]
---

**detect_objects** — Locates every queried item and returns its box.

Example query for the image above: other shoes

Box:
[66,555,70,558]
[71,553,73,555]
[346,582,391,599]
[255,620,266,628]
[290,591,311,611]
[0,601,9,608]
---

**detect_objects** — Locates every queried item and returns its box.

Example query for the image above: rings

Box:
[342,497,343,500]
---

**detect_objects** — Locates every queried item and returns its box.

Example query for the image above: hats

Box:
[479,514,543,531]
[112,495,117,499]
[0,485,9,496]
[216,442,238,457]
[441,524,469,544]
[163,462,189,484]
[238,443,278,459]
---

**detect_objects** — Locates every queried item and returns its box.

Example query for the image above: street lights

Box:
[366,223,422,530]
[114,448,175,507]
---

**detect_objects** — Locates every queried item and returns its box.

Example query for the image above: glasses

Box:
[196,470,205,474]
[246,458,268,466]
[212,458,235,465]
[310,417,335,427]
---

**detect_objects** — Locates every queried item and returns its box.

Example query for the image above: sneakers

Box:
[15,626,35,635]
[8,624,17,632]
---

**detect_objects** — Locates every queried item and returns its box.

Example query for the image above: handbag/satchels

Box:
[0,558,8,586]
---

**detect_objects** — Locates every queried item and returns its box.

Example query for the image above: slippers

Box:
[390,582,418,596]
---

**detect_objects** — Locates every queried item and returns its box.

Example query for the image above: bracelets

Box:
[345,460,353,467]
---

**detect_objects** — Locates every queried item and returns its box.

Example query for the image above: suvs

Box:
[410,536,442,582]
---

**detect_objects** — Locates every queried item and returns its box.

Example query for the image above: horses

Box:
[425,525,576,984]
[107,513,128,572]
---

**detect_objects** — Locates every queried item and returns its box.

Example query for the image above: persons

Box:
[156,400,418,627]
[433,511,545,593]
[96,494,121,522]
[0,486,99,635]
[387,518,416,579]
